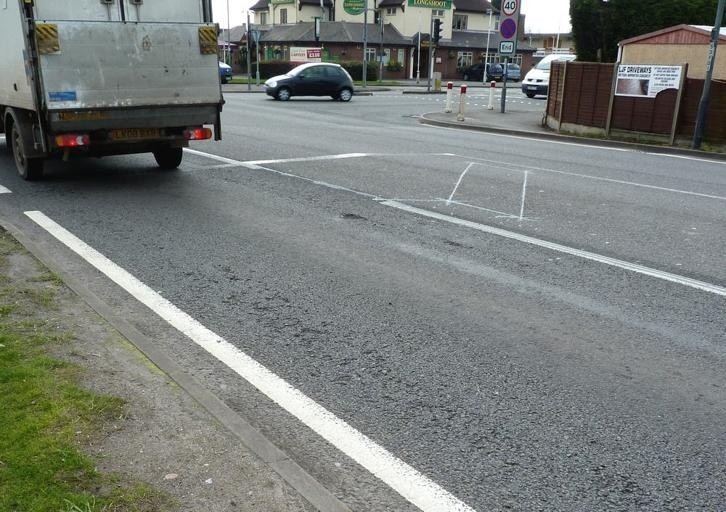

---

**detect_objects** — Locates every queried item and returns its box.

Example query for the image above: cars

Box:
[463,62,521,83]
[522,53,578,98]
[264,62,354,102]
[219,59,233,83]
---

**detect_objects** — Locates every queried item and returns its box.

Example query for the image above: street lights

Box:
[482,8,493,83]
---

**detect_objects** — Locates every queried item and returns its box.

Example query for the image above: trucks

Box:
[0,0,225,181]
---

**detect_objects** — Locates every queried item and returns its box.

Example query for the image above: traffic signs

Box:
[503,0,518,16]
